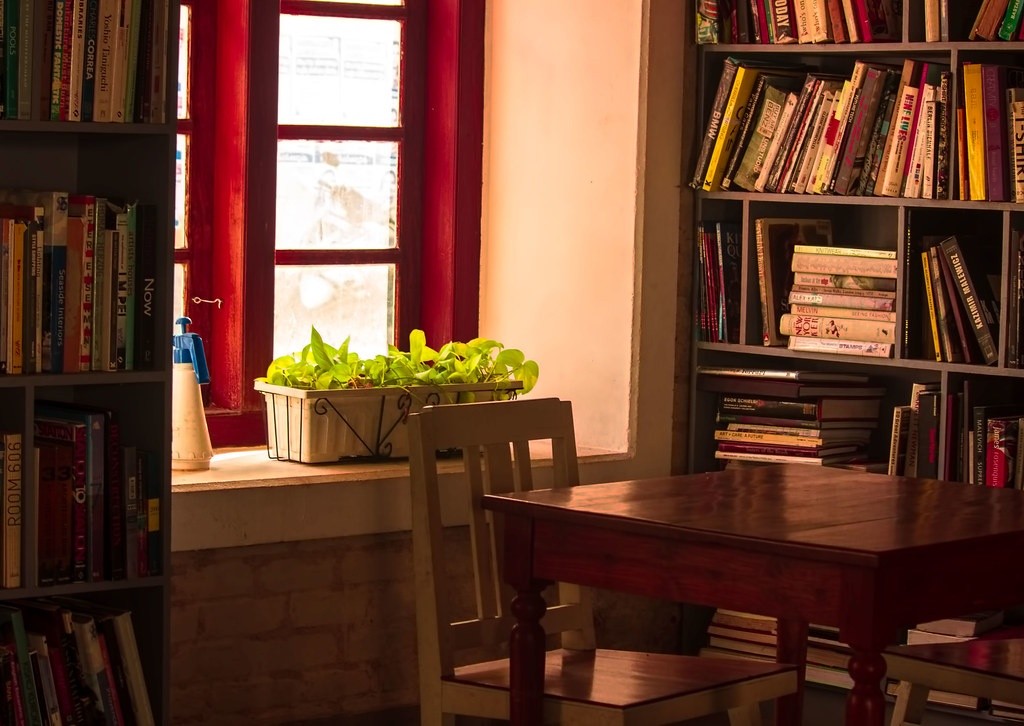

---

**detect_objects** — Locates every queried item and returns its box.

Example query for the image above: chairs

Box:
[880,626,1024,726]
[407,398,797,726]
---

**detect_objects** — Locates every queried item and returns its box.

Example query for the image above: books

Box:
[1,595,160,725]
[696,362,942,479]
[35,397,163,589]
[695,1,1024,44]
[1005,226,1024,369]
[693,606,856,691]
[903,208,1000,365]
[0,0,171,122]
[693,217,746,344]
[688,56,1024,203]
[0,184,158,375]
[947,379,1024,490]
[754,216,899,358]
[884,608,1024,719]
[0,424,25,590]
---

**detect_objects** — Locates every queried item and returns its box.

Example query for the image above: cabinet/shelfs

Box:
[0,0,180,726]
[680,0,1024,726]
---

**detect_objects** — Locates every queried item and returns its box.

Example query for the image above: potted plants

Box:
[254,324,539,464]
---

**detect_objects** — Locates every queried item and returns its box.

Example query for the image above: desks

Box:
[481,464,1024,726]
